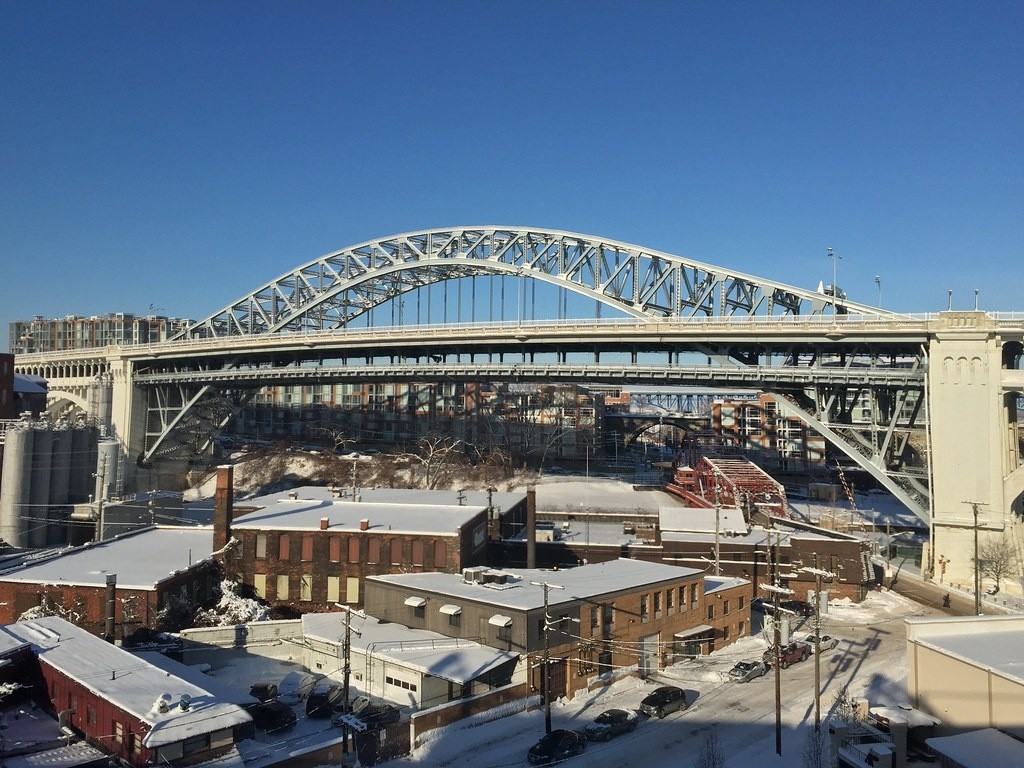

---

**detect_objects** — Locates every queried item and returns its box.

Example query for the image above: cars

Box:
[246,700,297,736]
[528,729,590,766]
[345,702,401,735]
[639,686,687,719]
[276,670,317,706]
[751,598,776,615]
[306,683,344,720]
[330,695,370,727]
[728,655,771,683]
[779,600,815,616]
[365,448,379,453]
[800,632,837,655]
[583,708,638,742]
[248,682,278,703]
[306,674,332,697]
[868,488,889,495]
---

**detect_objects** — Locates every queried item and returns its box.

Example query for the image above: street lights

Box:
[875,276,881,309]
[827,247,843,326]
[148,304,166,350]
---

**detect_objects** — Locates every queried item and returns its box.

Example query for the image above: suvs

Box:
[763,640,811,669]
